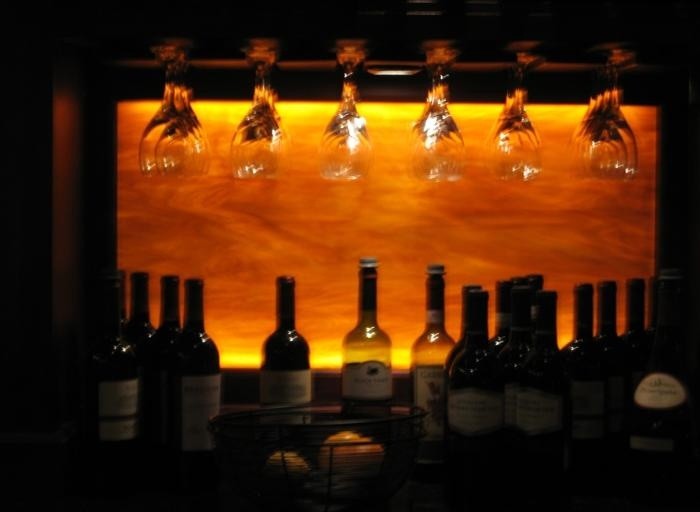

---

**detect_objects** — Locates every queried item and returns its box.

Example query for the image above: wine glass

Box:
[489,37,551,182]
[228,36,288,183]
[408,38,466,183]
[312,36,374,181]
[139,34,210,183]
[408,263,682,443]
[571,36,640,185]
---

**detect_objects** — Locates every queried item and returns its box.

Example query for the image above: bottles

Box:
[92,269,220,453]
[340,258,394,403]
[258,274,311,403]
